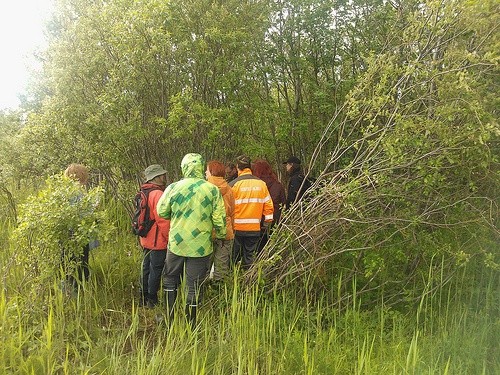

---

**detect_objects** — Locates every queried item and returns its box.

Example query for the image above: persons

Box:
[204,156,286,285]
[61,163,101,290]
[282,156,311,211]
[157,152,226,331]
[138,164,170,306]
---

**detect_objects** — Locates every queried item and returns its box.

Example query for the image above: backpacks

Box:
[131,188,164,238]
[298,175,324,201]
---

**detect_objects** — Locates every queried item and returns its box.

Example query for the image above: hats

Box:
[283,157,301,164]
[143,165,168,184]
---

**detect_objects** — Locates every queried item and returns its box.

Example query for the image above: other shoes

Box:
[148,298,158,309]
[139,298,148,307]
